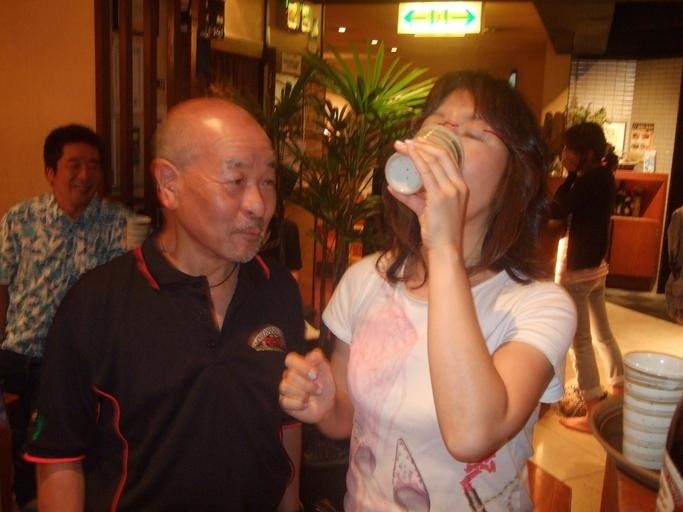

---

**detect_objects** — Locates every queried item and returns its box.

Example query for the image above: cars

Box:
[125,216,149,246]
[386,122,465,194]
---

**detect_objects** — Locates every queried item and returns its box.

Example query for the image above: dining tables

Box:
[285,34,441,510]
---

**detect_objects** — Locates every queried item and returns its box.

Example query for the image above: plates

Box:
[547,169,668,291]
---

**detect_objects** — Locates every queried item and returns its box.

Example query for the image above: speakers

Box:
[560,377,624,433]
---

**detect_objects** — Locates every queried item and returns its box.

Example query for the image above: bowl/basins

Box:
[207,262,239,290]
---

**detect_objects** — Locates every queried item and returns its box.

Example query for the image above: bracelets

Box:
[621,349,681,471]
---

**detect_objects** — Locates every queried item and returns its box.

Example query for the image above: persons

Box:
[273,68,579,511]
[22,93,303,512]
[546,125,628,434]
[1,124,134,511]
